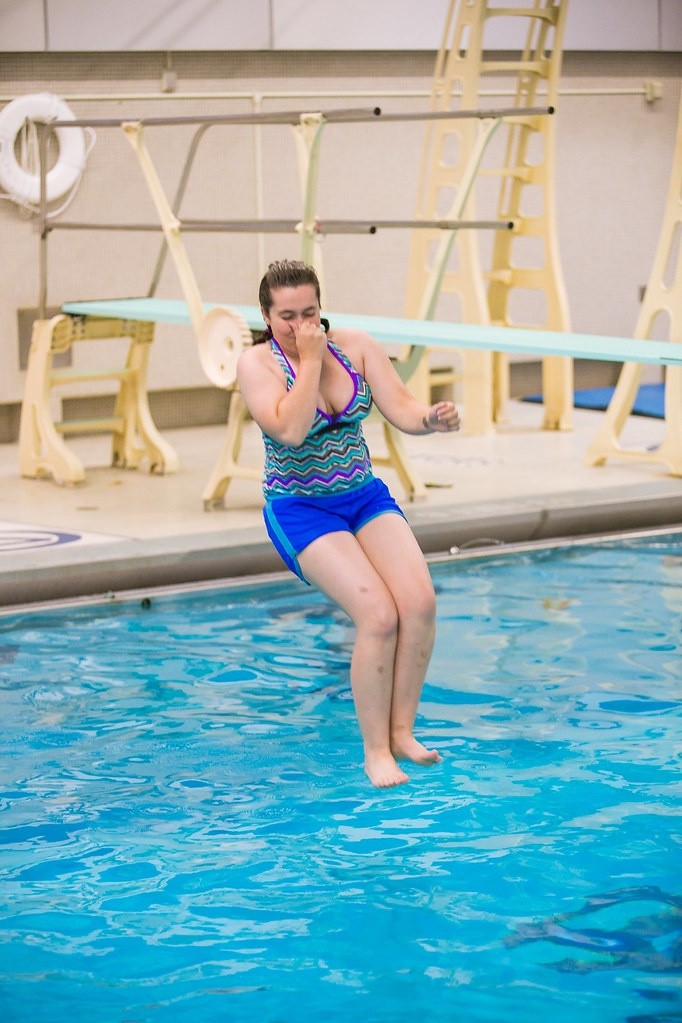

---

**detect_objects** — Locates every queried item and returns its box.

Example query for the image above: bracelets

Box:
[422,417,430,429]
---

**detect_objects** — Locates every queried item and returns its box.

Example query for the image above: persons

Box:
[236,258,461,785]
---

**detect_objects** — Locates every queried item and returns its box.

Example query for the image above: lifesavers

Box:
[0,91,87,206]
